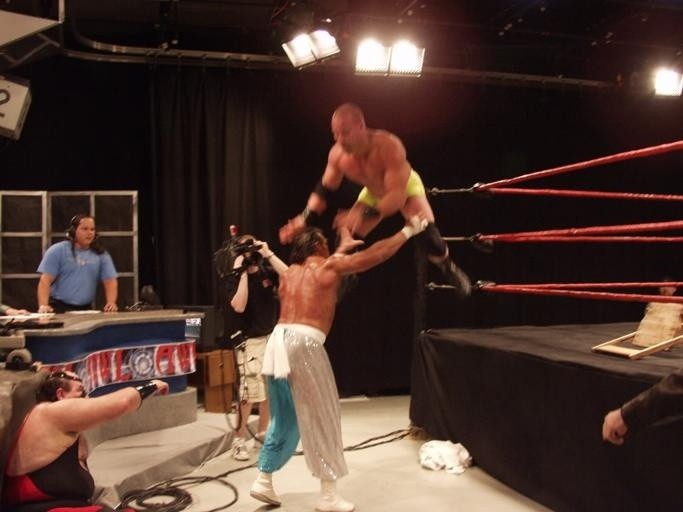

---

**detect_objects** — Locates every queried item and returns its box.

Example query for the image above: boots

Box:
[434,255,470,297]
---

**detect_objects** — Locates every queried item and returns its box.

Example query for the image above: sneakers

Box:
[314,479,354,512]
[249,471,282,506]
[232,438,249,461]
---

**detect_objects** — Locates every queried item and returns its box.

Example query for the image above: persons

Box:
[657,278,676,296]
[0,369,171,511]
[278,101,471,300]
[246,211,434,511]
[35,212,119,313]
[220,232,289,463]
[599,365,683,447]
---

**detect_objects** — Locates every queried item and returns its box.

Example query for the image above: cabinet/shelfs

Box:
[1,189,139,313]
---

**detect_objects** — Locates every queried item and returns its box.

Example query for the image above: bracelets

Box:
[262,250,274,262]
[300,208,320,228]
[362,206,380,219]
[135,380,158,400]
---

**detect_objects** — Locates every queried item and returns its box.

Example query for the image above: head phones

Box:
[65,215,99,240]
